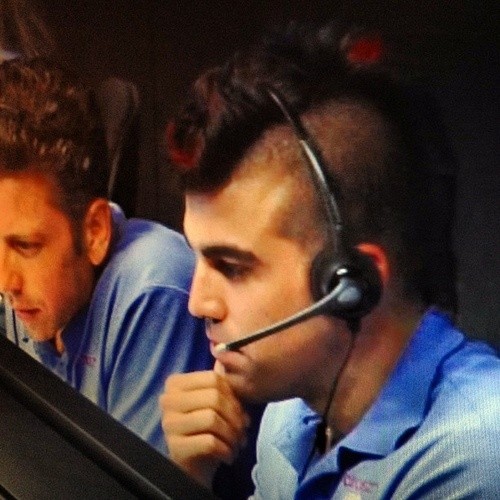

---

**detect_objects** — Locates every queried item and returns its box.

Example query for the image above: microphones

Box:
[214,278,361,354]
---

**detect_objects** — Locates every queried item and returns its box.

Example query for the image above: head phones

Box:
[263,84,381,323]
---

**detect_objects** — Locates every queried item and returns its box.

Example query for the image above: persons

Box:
[0,52,216,458]
[159,36,499,500]
